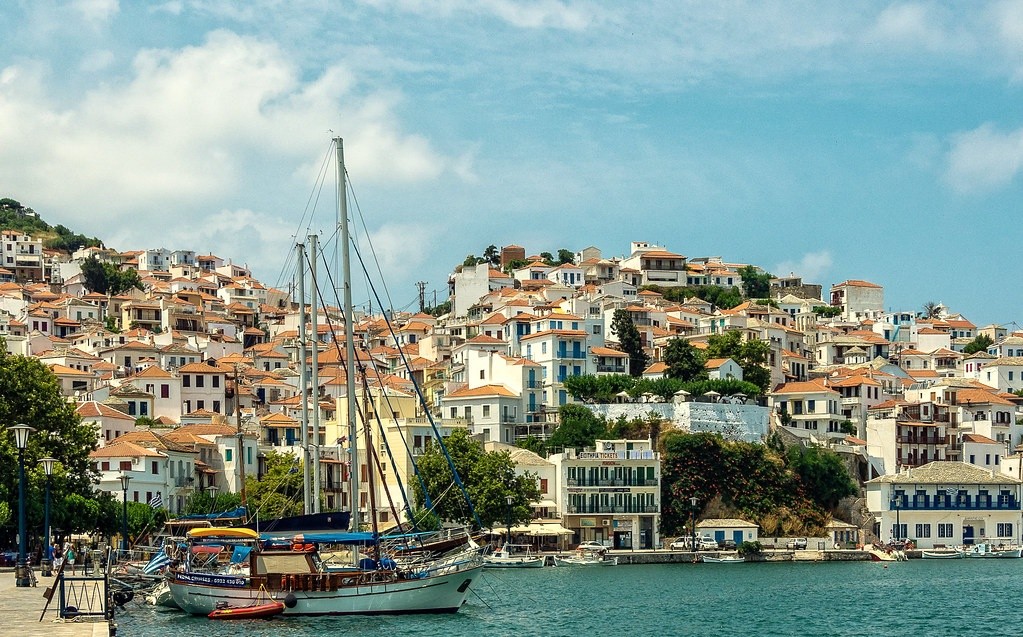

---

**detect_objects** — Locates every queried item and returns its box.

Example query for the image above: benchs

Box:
[83,557,107,576]
[933,544,947,550]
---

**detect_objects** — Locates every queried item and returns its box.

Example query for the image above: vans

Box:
[786,538,807,550]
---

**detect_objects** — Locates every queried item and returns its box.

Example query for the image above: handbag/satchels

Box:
[71,550,77,559]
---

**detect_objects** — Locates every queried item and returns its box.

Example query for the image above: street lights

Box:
[689,496,701,553]
[3,422,38,586]
[115,473,133,560]
[892,496,904,541]
[37,456,62,579]
[504,493,516,554]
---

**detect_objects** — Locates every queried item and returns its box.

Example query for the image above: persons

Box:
[889,537,893,544]
[905,537,911,547]
[53,544,106,575]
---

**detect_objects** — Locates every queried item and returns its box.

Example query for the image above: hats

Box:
[53,544,59,548]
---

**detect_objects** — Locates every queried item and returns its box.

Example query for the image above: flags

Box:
[149,494,163,508]
[141,549,171,574]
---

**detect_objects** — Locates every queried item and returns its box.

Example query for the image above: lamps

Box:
[101,434,105,439]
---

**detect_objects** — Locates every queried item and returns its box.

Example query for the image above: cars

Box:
[578,541,608,554]
[719,540,737,552]
[697,537,720,551]
[670,536,698,550]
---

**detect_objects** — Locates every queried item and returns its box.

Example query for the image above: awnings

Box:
[484,523,575,535]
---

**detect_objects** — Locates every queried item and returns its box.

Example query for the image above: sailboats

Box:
[121,134,490,612]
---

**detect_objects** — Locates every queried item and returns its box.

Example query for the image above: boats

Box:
[553,551,620,567]
[207,602,286,621]
[921,550,964,560]
[479,543,548,568]
[967,543,1023,559]
[702,555,745,563]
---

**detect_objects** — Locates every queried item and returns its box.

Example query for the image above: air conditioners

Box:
[592,358,598,363]
[131,457,138,465]
[602,520,611,527]
